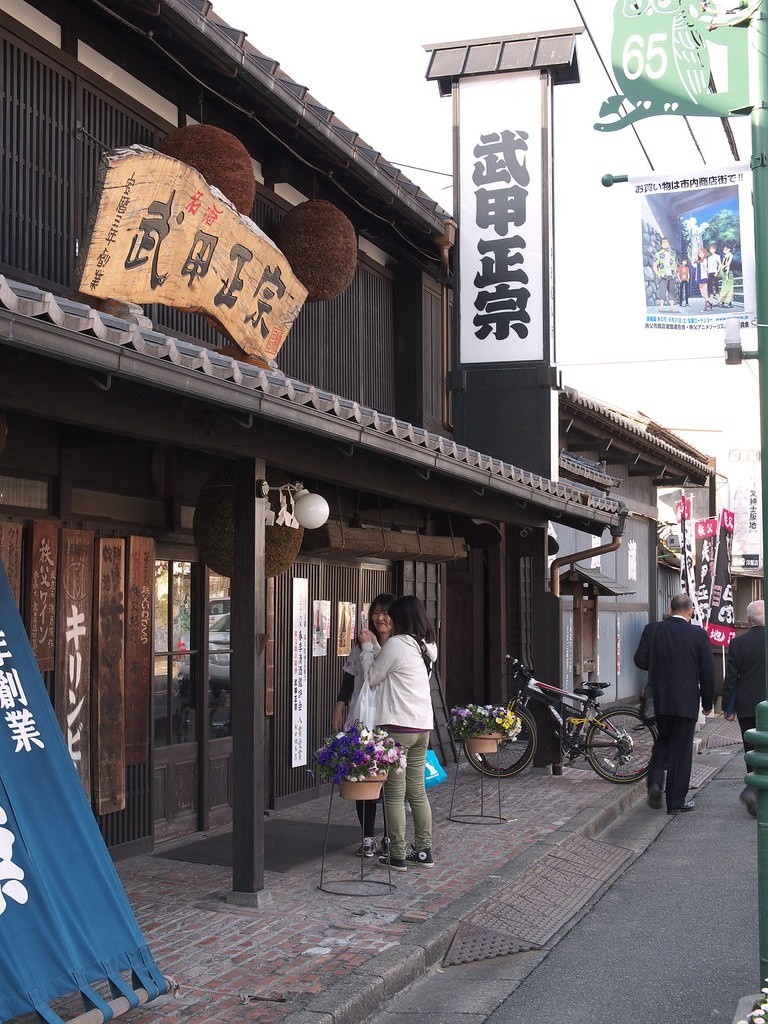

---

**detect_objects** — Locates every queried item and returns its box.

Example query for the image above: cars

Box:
[177,596,231,692]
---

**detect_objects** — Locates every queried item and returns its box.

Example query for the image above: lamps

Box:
[256,479,330,529]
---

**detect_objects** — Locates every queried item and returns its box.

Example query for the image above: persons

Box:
[358,595,437,871]
[332,594,399,857]
[634,594,715,814]
[722,600,766,818]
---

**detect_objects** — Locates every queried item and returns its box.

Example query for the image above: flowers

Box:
[446,703,522,745]
[306,719,407,782]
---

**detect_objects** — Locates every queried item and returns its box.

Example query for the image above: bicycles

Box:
[462,653,658,784]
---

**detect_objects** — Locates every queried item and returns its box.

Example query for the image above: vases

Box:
[341,775,387,800]
[467,733,502,753]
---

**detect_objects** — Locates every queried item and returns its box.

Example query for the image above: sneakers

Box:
[375,855,408,872]
[406,848,435,869]
[380,838,389,856]
[357,837,378,858]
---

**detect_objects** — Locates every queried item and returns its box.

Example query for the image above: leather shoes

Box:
[648,782,663,809]
[666,800,695,815]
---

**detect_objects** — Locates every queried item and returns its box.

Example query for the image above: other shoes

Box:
[741,787,758,818]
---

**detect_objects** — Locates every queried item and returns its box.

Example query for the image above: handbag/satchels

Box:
[423,750,448,790]
